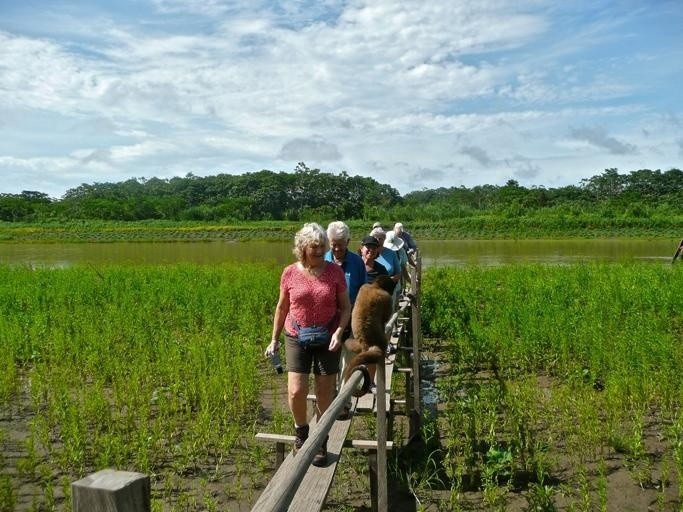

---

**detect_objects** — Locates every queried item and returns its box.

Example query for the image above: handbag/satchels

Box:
[272,338,280,341]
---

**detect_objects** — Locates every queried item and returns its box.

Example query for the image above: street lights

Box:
[297,324,329,353]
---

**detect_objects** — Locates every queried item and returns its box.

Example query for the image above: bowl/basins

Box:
[306,267,317,276]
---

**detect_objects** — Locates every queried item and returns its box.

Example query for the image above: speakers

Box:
[293,424,309,457]
[311,435,329,467]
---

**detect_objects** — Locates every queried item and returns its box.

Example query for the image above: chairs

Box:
[335,409,350,420]
[384,334,399,364]
[368,383,377,395]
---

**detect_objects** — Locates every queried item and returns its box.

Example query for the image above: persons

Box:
[355,219,417,391]
[261,221,351,468]
[320,219,367,421]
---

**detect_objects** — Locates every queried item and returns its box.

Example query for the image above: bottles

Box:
[383,231,404,251]
[360,236,380,247]
